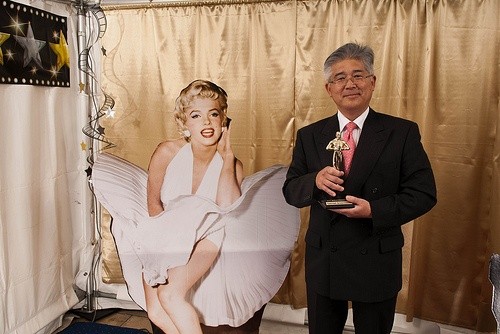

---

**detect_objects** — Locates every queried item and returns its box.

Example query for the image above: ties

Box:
[342,122,358,177]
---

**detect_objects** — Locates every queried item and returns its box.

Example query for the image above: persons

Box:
[282,42,438,334]
[92,79,301,334]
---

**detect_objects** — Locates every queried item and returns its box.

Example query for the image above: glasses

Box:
[329,74,370,86]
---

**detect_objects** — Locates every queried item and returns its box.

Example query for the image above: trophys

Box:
[325,132,353,202]
